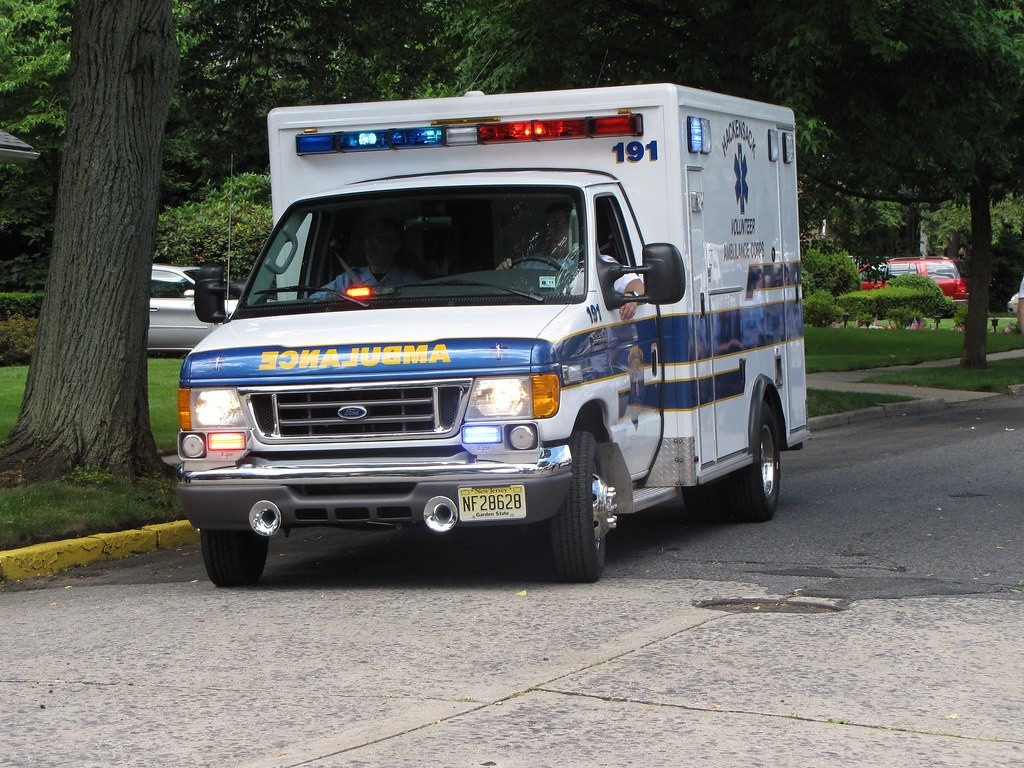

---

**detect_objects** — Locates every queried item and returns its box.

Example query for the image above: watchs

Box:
[624,290,640,299]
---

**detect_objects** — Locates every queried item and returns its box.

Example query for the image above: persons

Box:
[496,202,650,320]
[308,219,446,299]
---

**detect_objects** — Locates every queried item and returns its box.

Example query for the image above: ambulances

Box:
[174,83,814,588]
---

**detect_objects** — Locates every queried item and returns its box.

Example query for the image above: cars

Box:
[1007,293,1021,314]
[146,262,276,356]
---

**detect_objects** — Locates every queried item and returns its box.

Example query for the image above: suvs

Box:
[858,255,970,308]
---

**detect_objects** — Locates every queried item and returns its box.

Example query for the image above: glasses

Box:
[546,221,570,228]
